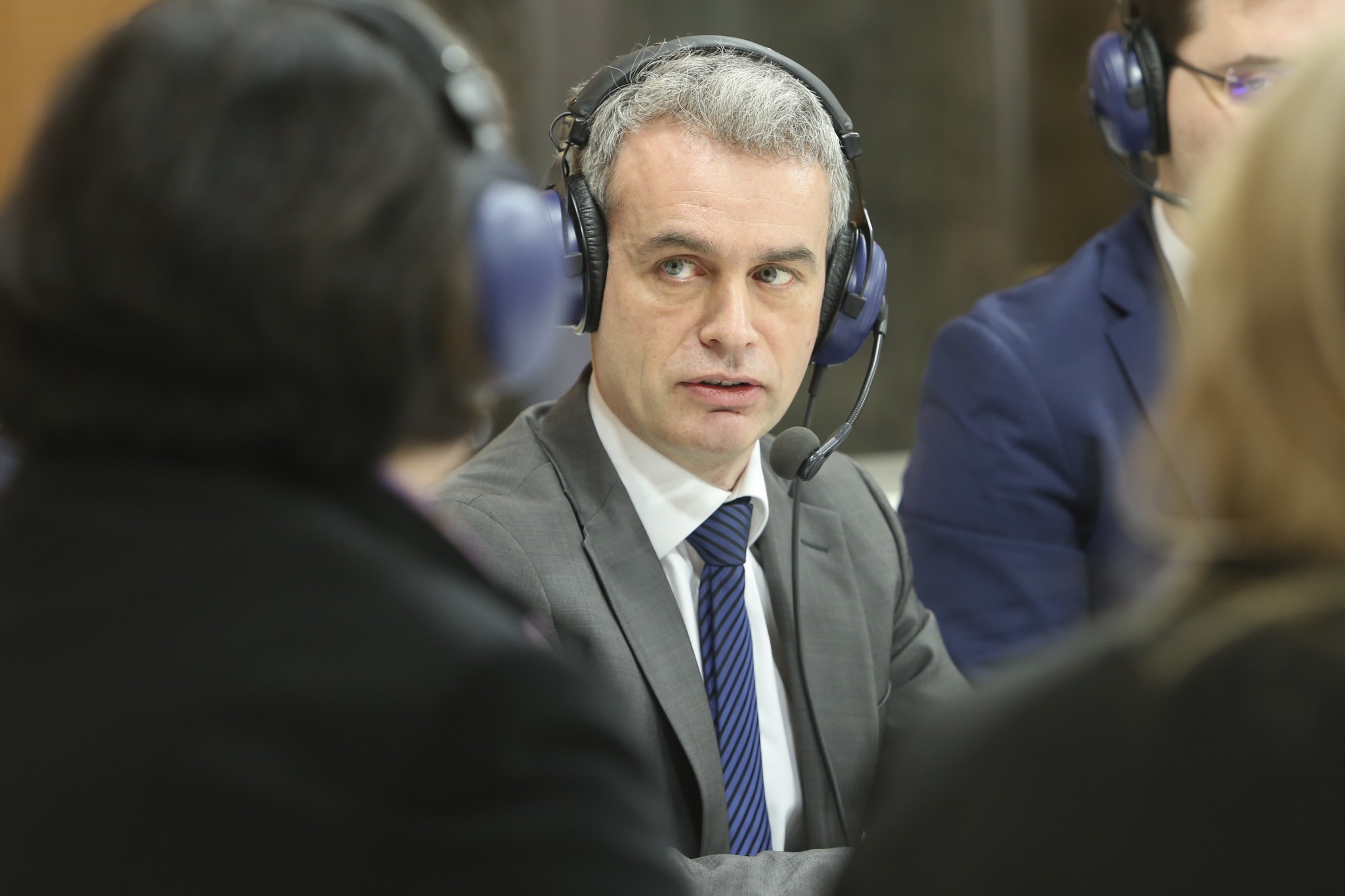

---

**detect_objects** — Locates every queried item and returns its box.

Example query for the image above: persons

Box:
[878,0,1345,695]
[438,32,1007,893]
[0,1,703,896]
[811,37,1345,896]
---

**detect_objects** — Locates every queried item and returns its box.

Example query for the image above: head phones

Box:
[1085,0,1173,165]
[326,0,560,411]
[530,34,887,369]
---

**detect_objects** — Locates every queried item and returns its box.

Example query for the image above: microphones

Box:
[769,422,859,482]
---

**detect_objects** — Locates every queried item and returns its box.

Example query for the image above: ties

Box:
[685,495,773,858]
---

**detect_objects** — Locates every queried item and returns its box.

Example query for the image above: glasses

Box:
[1157,41,1281,101]
